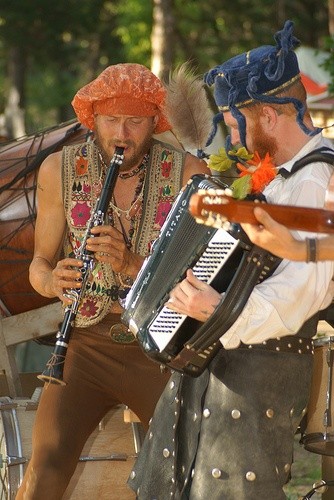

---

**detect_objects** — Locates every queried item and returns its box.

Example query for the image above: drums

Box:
[1,114,97,345]
[301,332,332,457]
[0,396,144,499]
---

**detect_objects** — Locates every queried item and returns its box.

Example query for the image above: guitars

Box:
[189,186,334,235]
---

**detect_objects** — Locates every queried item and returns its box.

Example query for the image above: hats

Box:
[71,63,171,132]
[203,18,334,169]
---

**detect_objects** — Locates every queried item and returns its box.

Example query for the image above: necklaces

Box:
[97,151,150,288]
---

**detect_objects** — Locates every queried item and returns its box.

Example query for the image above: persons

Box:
[15,64,213,500]
[240,206,334,261]
[126,44,334,500]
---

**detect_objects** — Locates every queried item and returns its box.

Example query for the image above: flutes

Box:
[38,145,124,387]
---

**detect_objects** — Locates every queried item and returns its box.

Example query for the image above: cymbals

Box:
[297,479,333,500]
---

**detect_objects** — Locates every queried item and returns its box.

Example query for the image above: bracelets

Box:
[306,238,316,264]
